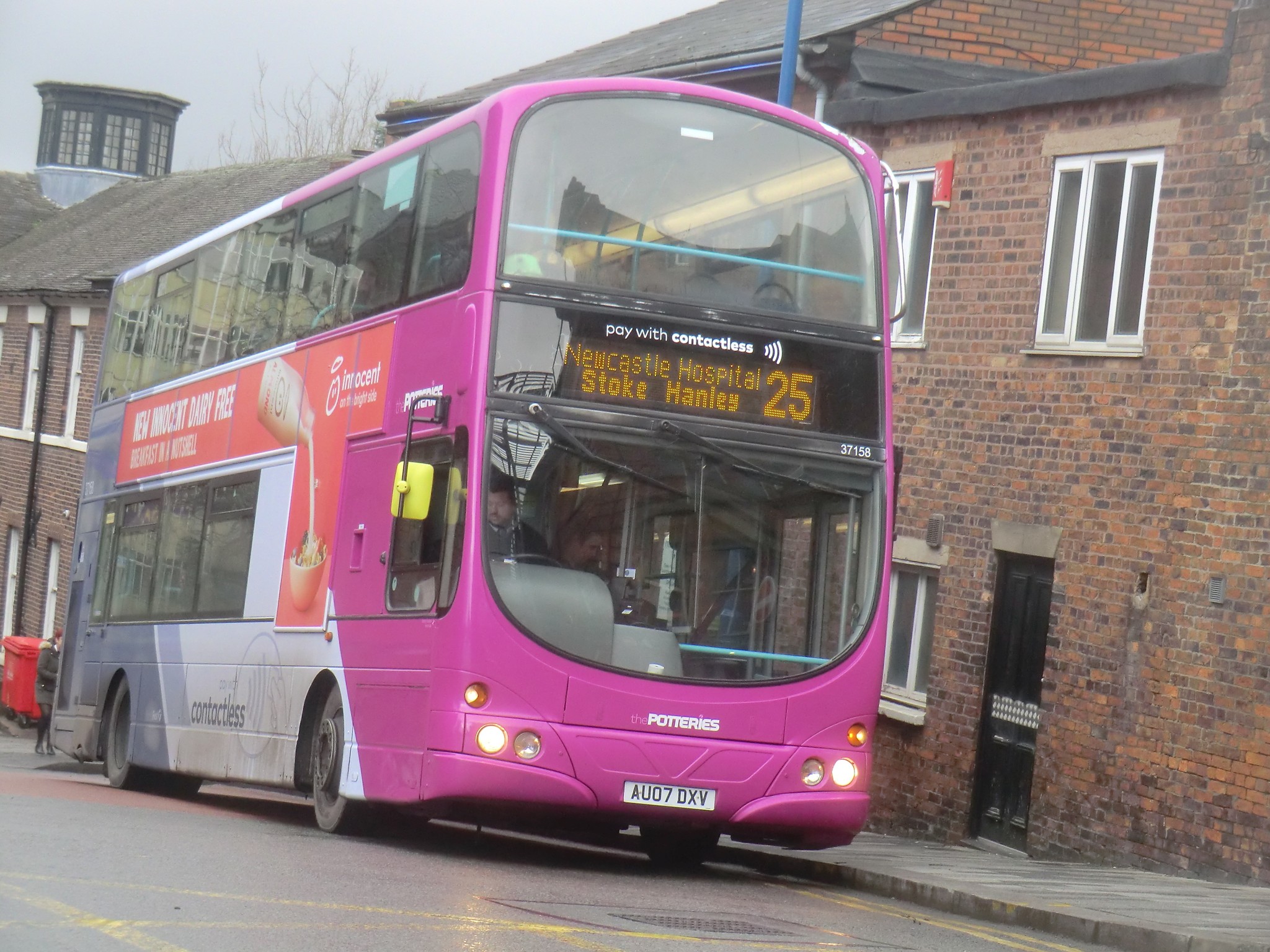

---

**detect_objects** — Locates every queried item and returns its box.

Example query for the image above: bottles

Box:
[257,356,315,449]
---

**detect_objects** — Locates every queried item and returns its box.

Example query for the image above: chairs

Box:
[508,252,798,308]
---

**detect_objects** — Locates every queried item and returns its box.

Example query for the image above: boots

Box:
[46,717,56,755]
[36,716,46,755]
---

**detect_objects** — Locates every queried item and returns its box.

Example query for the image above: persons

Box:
[486,474,547,564]
[35,628,62,756]
[546,512,611,585]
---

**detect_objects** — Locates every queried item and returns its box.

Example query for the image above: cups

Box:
[288,547,328,612]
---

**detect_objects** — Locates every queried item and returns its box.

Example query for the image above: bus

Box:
[48,74,906,879]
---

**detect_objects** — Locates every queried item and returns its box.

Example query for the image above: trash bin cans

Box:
[0,636,48,729]
